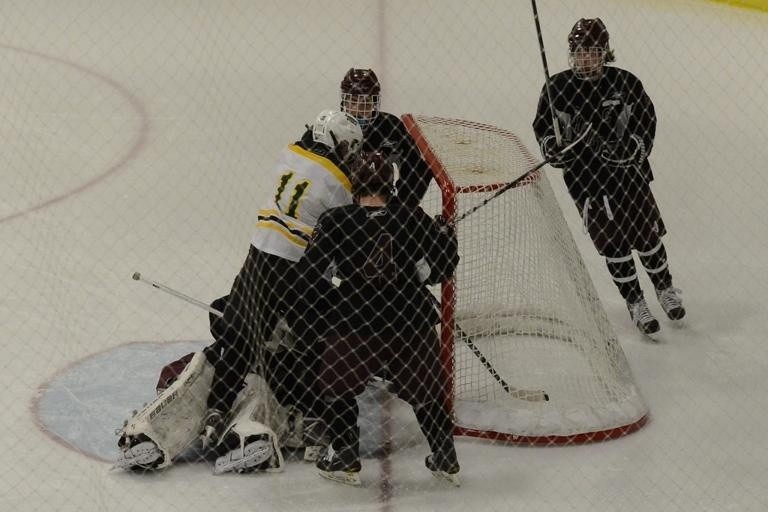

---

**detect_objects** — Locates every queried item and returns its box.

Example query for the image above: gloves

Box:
[598,133,644,168]
[539,135,576,168]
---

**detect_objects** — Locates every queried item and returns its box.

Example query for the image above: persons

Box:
[531,17,687,335]
[111,67,433,476]
[295,142,461,488]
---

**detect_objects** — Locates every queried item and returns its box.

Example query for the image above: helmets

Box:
[569,17,609,82]
[312,67,400,197]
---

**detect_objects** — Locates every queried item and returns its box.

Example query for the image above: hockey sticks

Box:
[448,122,592,229]
[425,287,549,404]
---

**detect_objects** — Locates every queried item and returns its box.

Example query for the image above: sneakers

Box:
[115,441,158,474]
[317,450,360,471]
[626,297,660,335]
[217,440,274,474]
[425,454,460,475]
[654,275,685,320]
